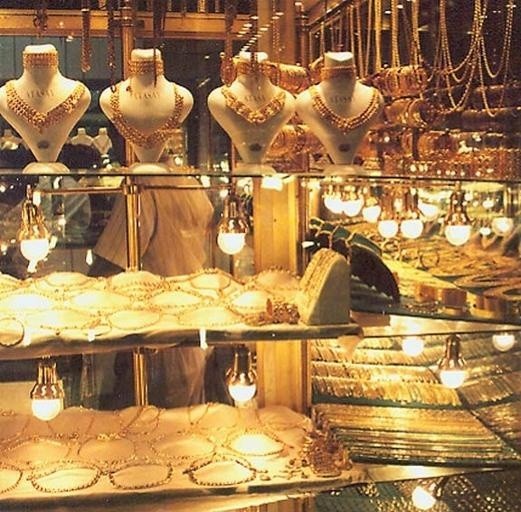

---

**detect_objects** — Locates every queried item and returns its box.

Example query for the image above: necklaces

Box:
[0,0,520,493]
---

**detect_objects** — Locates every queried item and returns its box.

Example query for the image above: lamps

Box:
[318,175,520,245]
[28,357,68,420]
[225,343,259,404]
[213,182,254,256]
[13,184,54,265]
[437,334,471,391]
[410,476,449,510]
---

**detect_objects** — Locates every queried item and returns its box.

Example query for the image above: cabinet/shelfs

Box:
[1,161,373,512]
[306,163,519,510]
